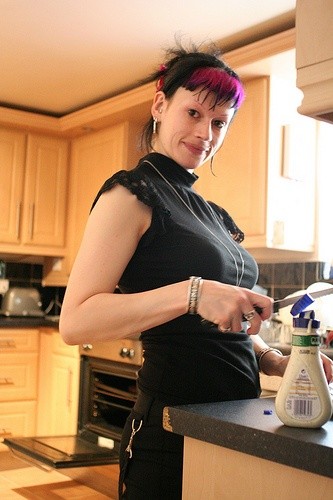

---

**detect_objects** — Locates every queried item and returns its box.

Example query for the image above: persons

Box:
[57,33,333,500]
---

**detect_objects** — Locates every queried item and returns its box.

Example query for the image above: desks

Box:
[163,397,333,500]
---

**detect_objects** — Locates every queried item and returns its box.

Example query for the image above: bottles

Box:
[275,294,333,428]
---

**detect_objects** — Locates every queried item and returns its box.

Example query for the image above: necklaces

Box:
[143,160,245,287]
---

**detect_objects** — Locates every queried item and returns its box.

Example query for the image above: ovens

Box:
[2,333,144,471]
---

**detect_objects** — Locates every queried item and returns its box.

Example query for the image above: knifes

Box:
[201,287,333,329]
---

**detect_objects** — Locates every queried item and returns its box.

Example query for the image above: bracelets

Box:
[256,348,283,376]
[186,276,203,315]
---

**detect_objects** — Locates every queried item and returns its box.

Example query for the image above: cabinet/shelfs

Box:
[0,327,142,440]
[1,120,136,287]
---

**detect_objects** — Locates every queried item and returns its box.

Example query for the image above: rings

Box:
[243,309,256,320]
